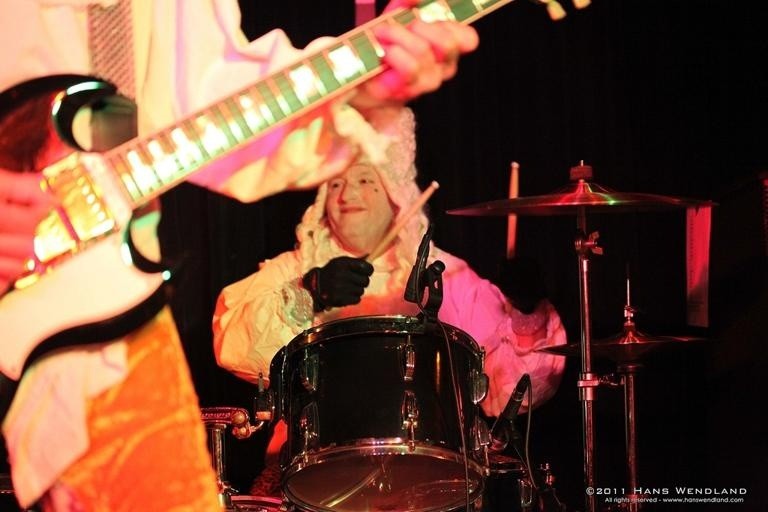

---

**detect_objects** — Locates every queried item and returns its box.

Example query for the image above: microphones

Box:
[404,234,430,303]
[487,373,530,454]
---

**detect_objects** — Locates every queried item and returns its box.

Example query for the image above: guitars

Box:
[1,0,593,425]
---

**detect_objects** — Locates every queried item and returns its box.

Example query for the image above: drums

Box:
[268,315,484,512]
[198,404,294,509]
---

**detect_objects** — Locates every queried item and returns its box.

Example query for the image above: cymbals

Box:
[527,333,710,365]
[445,194,717,215]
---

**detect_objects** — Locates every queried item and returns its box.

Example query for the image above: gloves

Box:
[303,256,375,313]
[503,260,546,313]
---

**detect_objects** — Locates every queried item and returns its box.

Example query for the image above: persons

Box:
[0,0,482,512]
[208,105,568,419]
